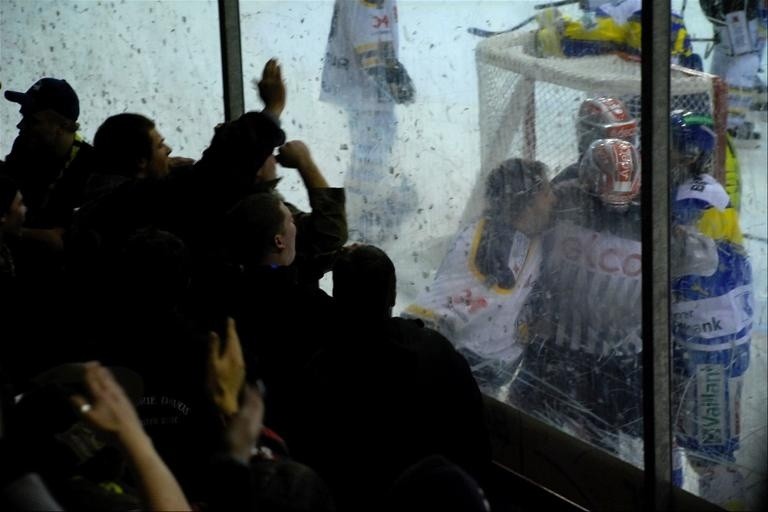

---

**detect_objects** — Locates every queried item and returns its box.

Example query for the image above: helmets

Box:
[575,98,641,204]
[670,109,717,162]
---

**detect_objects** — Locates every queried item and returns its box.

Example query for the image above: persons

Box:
[0,54,500,511]
[500,0,767,511]
[315,0,422,243]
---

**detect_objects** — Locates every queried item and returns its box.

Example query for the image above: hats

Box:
[5,78,79,119]
